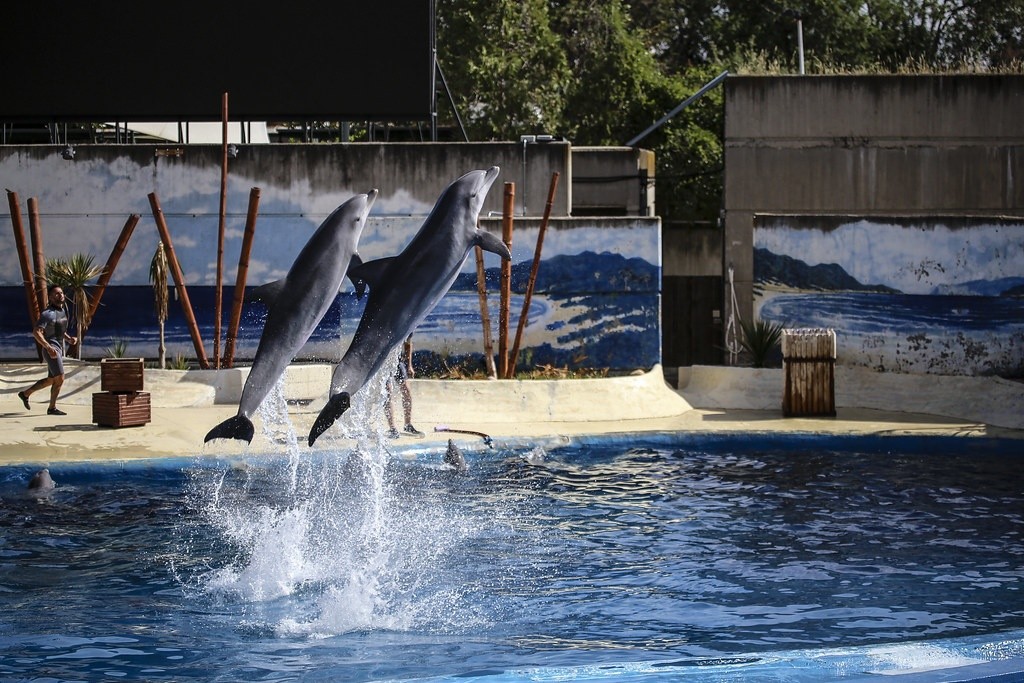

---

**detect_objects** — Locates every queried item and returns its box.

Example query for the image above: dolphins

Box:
[307,165,513,448]
[28,467,56,490]
[443,438,467,470]
[203,187,378,449]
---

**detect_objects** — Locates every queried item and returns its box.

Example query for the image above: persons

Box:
[382,331,420,439]
[18,286,77,416]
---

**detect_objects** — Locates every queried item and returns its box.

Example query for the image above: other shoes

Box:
[404,424,419,433]
[47,408,67,415]
[390,429,399,438]
[18,391,31,410]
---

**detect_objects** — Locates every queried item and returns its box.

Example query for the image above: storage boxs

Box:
[91,393,151,428]
[100,358,144,390]
